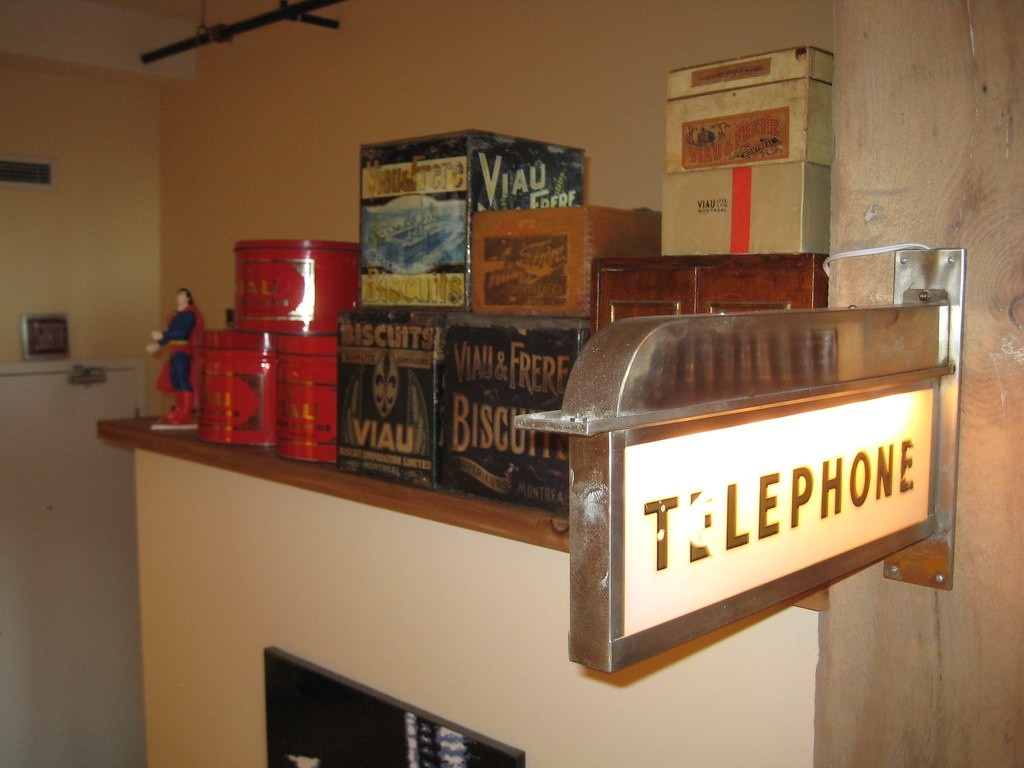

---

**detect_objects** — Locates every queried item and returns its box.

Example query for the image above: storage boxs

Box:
[335,47,836,515]
